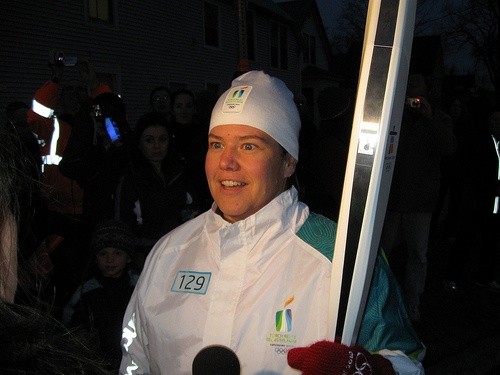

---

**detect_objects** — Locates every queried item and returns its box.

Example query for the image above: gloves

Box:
[288,341,395,375]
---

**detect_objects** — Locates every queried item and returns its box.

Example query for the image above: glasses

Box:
[152,95,169,102]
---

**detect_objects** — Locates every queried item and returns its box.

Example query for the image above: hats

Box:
[92,219,133,253]
[207,70,301,161]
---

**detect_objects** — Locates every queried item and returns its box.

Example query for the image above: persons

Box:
[119,70,427,375]
[62,219,141,369]
[0,100,116,375]
[12,47,500,324]
